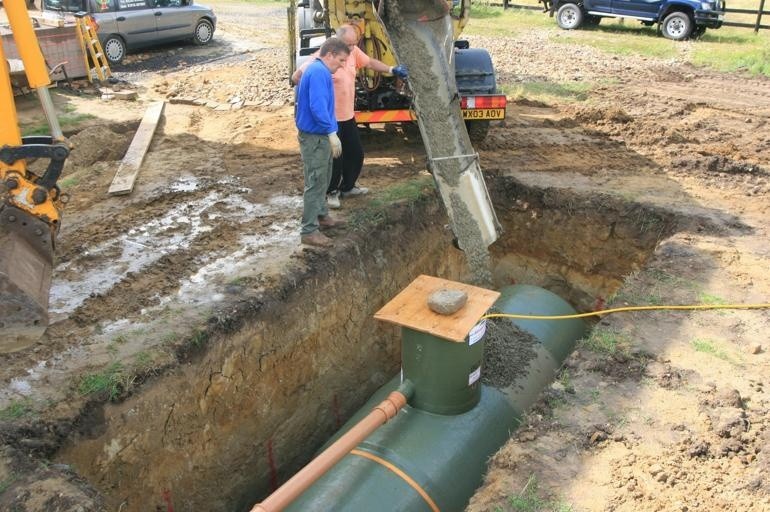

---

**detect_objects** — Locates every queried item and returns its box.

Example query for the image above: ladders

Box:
[73,11,113,84]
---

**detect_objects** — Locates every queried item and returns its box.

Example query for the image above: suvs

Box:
[538,0,725,40]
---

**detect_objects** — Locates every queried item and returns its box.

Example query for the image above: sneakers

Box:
[325,189,341,209]
[342,185,370,197]
[301,229,336,247]
[317,214,350,229]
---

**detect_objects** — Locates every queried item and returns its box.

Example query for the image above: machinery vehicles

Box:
[2,0,74,358]
[286,1,506,254]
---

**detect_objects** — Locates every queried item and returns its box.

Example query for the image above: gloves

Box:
[328,131,343,159]
[390,65,409,80]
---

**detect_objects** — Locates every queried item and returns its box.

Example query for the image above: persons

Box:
[301,38,351,246]
[291,24,408,211]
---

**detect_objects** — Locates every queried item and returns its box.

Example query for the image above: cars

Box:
[41,0,217,65]
